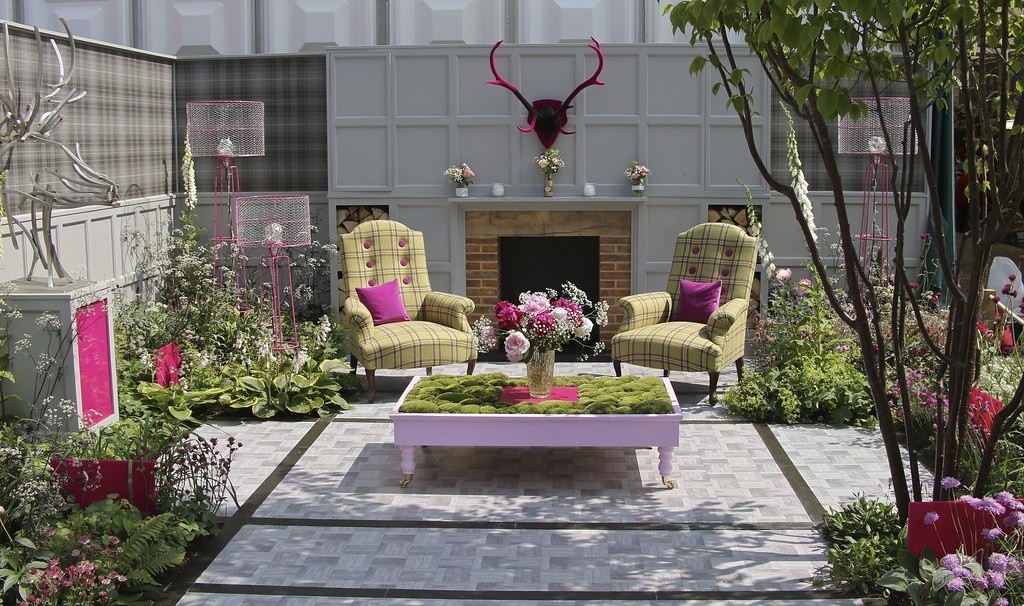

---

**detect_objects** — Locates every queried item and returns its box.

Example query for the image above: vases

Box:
[632,180,643,197]
[584,183,596,197]
[152,340,181,389]
[969,386,1002,439]
[524,348,554,398]
[543,172,554,197]
[492,183,504,196]
[908,499,1024,563]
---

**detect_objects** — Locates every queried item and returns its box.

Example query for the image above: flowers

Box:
[624,161,652,197]
[471,281,609,396]
[926,476,1024,606]
[534,149,566,172]
[145,300,167,313]
[444,163,475,188]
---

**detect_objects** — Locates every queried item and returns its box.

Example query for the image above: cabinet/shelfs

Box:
[456,187,468,198]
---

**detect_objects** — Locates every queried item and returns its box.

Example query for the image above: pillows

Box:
[677,279,722,324]
[355,278,411,325]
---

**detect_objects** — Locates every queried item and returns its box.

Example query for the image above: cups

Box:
[584,183,595,197]
[492,183,504,197]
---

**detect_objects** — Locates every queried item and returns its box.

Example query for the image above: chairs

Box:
[611,221,760,405]
[338,219,478,404]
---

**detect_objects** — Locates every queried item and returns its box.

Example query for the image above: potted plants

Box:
[50,417,188,513]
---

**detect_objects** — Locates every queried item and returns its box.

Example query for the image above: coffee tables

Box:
[389,376,684,489]
